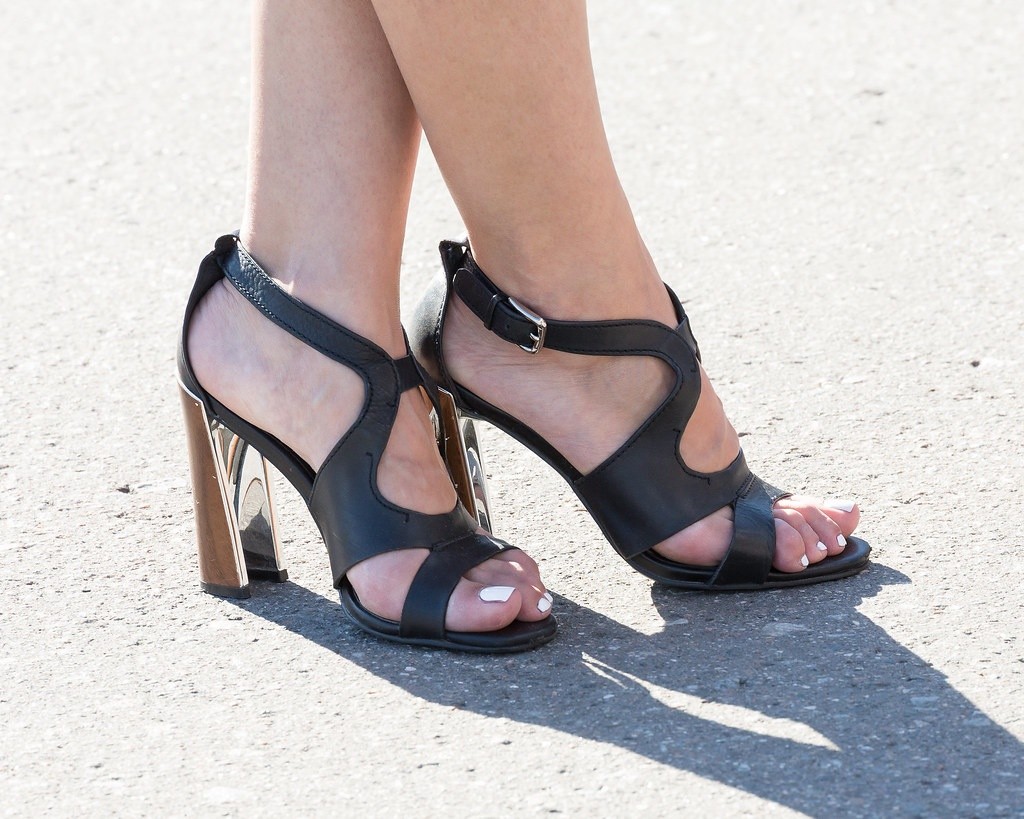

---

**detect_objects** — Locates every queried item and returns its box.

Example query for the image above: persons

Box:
[176,0,872,657]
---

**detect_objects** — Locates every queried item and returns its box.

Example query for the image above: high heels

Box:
[175,228,558,653]
[405,234,871,590]
